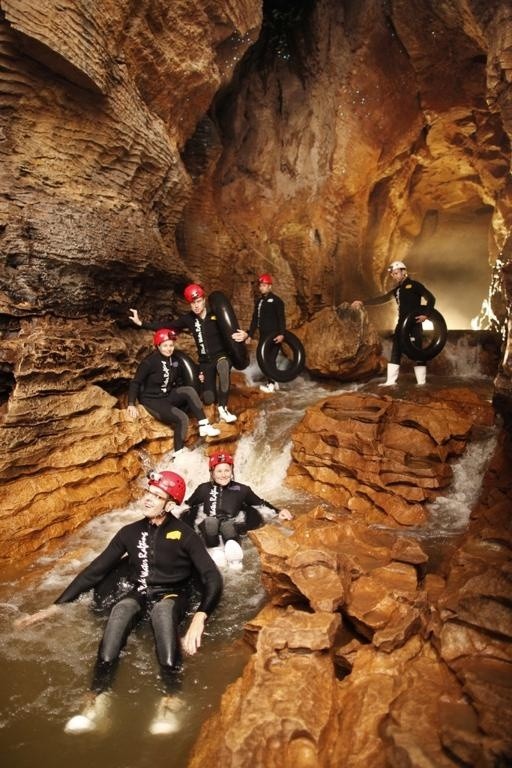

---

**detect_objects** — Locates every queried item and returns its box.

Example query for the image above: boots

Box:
[218,405,237,423]
[378,363,400,386]
[414,366,426,386]
[198,419,220,437]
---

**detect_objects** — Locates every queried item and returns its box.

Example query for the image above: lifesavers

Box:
[209,290,249,370]
[256,330,305,382]
[398,306,447,360]
[94,554,206,643]
[136,351,201,420]
[179,506,262,547]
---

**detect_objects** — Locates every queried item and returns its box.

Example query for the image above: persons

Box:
[122,328,220,454]
[172,450,294,569]
[243,273,286,394]
[128,283,248,424]
[347,260,436,388]
[18,466,223,738]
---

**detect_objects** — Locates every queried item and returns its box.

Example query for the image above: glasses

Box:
[145,488,166,500]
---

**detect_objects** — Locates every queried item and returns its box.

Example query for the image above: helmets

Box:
[147,468,187,506]
[209,450,234,468]
[256,275,272,284]
[153,328,177,346]
[387,260,406,273]
[184,284,206,302]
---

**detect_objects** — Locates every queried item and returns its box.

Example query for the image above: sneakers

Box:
[64,693,111,733]
[260,382,280,393]
[150,697,178,733]
[209,539,244,572]
